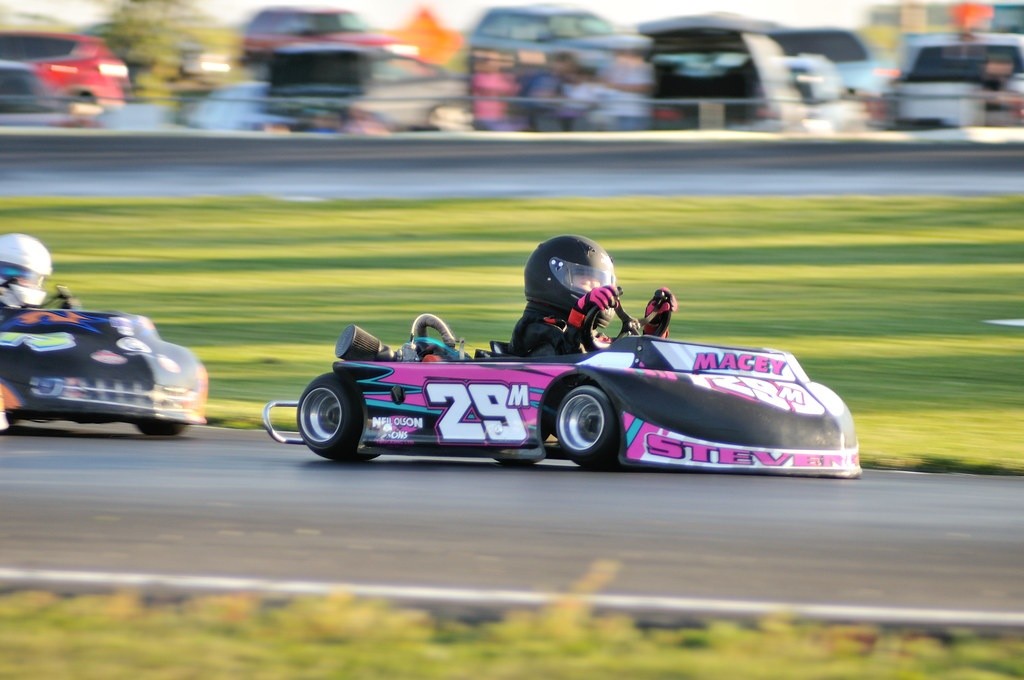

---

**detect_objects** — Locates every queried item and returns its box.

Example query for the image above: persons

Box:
[508,234,679,357]
[1,231,81,313]
[981,51,1024,128]
[470,48,579,132]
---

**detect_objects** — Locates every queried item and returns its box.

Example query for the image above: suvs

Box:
[465,5,655,91]
[272,39,466,130]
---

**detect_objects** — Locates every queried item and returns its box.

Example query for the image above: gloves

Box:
[643,288,677,338]
[566,285,621,331]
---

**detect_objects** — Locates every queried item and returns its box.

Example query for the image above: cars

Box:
[242,9,415,59]
[0,31,130,108]
[887,33,1024,125]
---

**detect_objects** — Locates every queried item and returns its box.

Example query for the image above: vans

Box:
[620,14,872,137]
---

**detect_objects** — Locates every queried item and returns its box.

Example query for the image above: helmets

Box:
[0,233,51,308]
[524,235,619,328]
